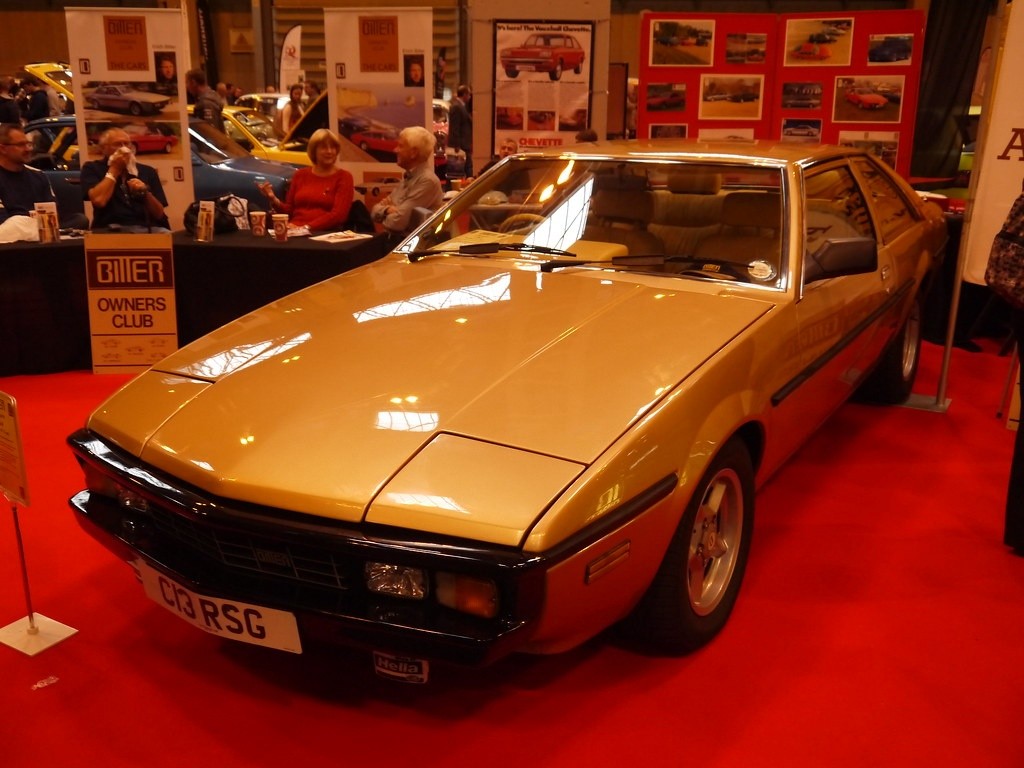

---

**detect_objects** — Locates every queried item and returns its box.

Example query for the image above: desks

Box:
[171,226,393,350]
[0,228,111,377]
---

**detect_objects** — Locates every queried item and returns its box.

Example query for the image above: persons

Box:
[80,127,171,231]
[254,128,354,233]
[304,80,321,110]
[575,129,599,144]
[217,82,227,103]
[0,121,90,231]
[368,126,444,261]
[447,85,473,177]
[0,74,50,124]
[477,138,531,198]
[185,67,227,155]
[226,84,235,105]
[282,83,308,134]
[405,58,425,88]
[156,54,177,84]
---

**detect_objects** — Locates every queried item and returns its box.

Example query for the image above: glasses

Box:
[3,140,32,149]
[102,140,130,148]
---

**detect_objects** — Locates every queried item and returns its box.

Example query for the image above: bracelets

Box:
[303,225,310,229]
[138,185,151,196]
[105,173,117,183]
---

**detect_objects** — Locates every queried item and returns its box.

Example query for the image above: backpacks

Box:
[983,191,1024,309]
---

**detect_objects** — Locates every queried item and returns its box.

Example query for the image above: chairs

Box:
[649,172,726,255]
[695,191,780,284]
[805,171,851,216]
[582,188,660,273]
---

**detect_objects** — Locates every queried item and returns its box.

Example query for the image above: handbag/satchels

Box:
[183,196,245,236]
[444,146,466,170]
[220,194,250,230]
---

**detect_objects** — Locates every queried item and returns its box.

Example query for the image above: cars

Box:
[234,91,469,168]
[22,61,314,169]
[785,124,820,138]
[846,86,889,111]
[64,137,948,683]
[500,32,585,82]
[706,90,732,102]
[787,18,851,63]
[653,21,712,48]
[726,92,760,104]
[783,94,821,109]
[93,122,178,153]
[22,114,299,235]
[86,85,170,117]
[497,112,522,128]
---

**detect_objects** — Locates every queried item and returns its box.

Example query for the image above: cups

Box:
[272,214,288,243]
[250,212,266,236]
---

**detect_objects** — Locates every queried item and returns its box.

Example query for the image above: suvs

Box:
[725,37,767,56]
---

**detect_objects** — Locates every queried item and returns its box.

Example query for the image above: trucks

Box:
[866,34,913,64]
